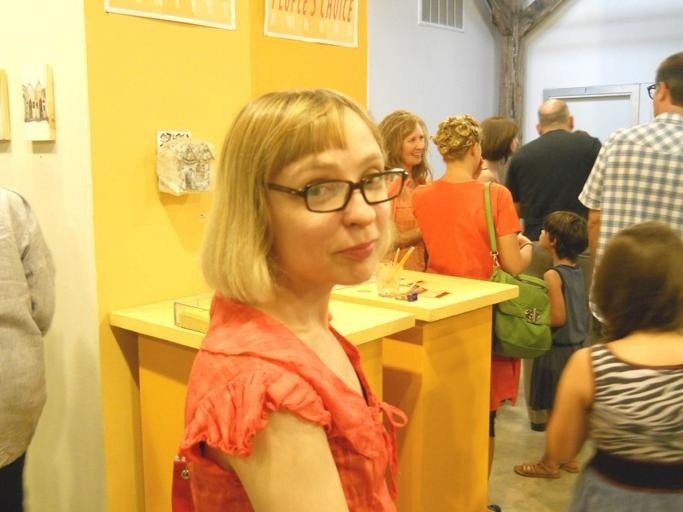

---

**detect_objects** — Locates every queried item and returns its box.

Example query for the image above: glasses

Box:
[648,84,656,99]
[268,165,408,212]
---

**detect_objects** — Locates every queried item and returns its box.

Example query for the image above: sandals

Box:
[514,461,577,478]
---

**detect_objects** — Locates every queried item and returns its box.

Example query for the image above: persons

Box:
[413,116,537,511]
[0,186,56,511]
[515,211,591,477]
[506,99,602,431]
[171,89,410,511]
[373,110,432,275]
[476,116,521,187]
[579,53,682,346]
[544,221,683,512]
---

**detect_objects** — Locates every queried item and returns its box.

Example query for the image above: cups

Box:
[374,261,402,298]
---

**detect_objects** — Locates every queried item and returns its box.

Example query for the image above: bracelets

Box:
[520,241,536,252]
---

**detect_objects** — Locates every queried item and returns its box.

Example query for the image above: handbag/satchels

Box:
[489,270,552,358]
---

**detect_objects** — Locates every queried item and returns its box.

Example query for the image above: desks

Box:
[110,293,415,512]
[330,269,519,512]
